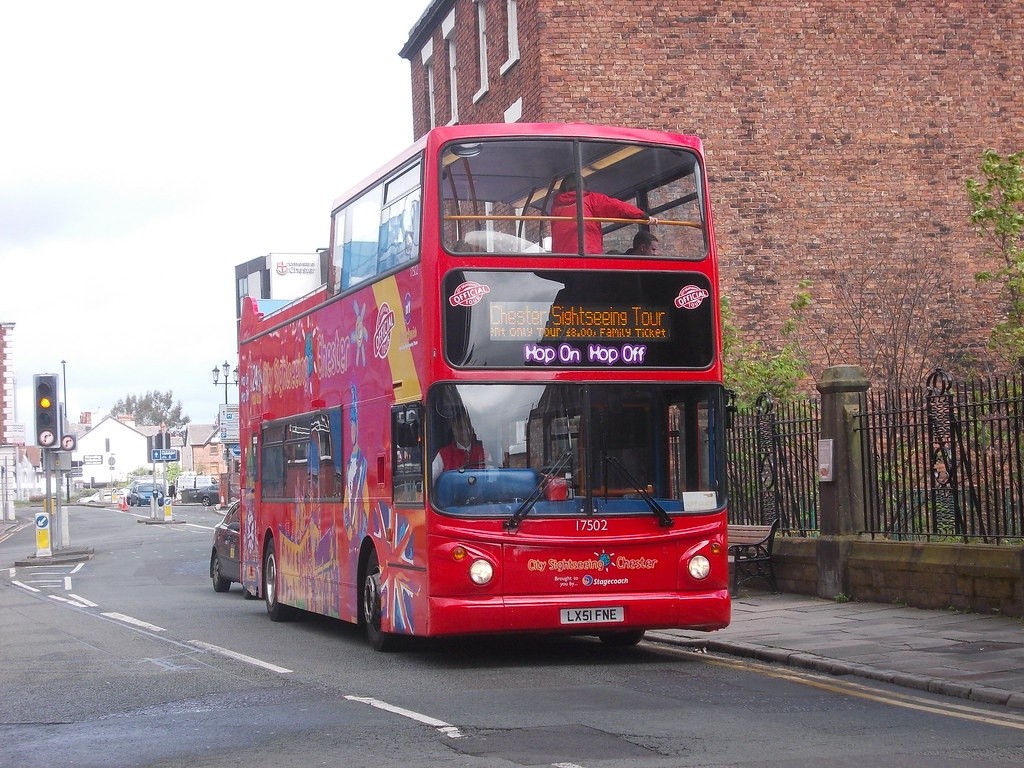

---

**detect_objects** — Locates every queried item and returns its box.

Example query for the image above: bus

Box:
[234,120,730,652]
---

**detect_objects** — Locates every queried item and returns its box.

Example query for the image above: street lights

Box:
[212,360,238,404]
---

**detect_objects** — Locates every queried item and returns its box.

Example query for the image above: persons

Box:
[168,482,175,497]
[549,173,658,256]
[620,231,658,256]
[431,409,496,488]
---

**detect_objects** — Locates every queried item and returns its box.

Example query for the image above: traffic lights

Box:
[34,373,59,448]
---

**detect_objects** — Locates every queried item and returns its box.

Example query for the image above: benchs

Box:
[728,520,784,598]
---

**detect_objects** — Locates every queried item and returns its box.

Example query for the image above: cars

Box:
[192,484,240,506]
[77,491,124,503]
[209,500,241,591]
[127,484,163,507]
